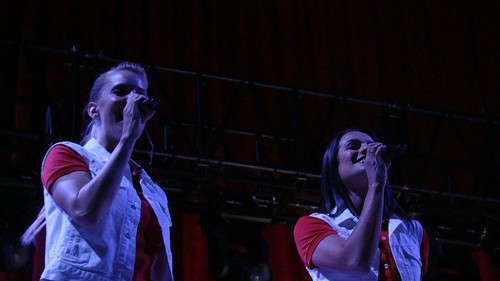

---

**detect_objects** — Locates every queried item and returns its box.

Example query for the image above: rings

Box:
[369,149,375,154]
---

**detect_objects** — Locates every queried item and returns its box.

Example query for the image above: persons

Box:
[31,63,178,281]
[291,128,434,281]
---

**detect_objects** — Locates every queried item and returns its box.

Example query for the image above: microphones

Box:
[379,144,407,161]
[138,98,162,115]
